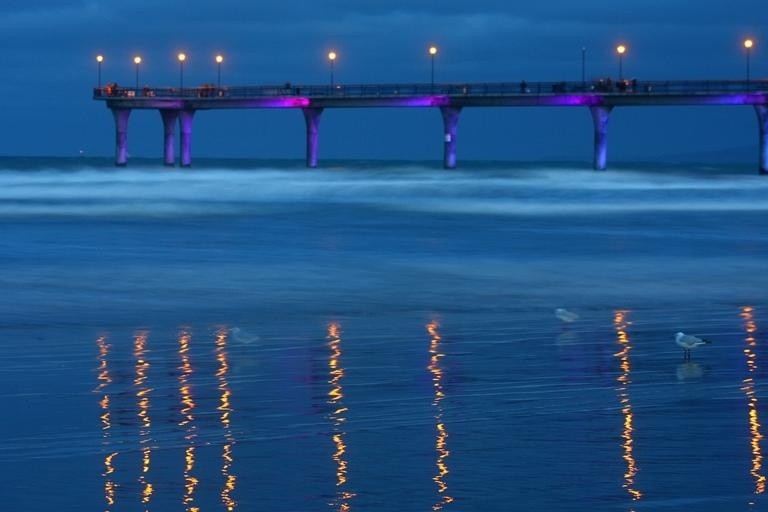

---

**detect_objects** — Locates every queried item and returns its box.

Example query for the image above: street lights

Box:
[744,39,753,81]
[216,55,223,84]
[428,47,437,83]
[328,52,336,84]
[96,55,103,87]
[178,54,186,88]
[581,45,587,81]
[134,56,141,88]
[618,45,625,78]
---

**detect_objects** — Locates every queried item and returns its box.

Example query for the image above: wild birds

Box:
[555,306,581,331]
[674,330,713,362]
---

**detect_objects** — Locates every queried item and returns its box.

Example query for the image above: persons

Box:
[521,77,637,93]
[104,82,224,97]
